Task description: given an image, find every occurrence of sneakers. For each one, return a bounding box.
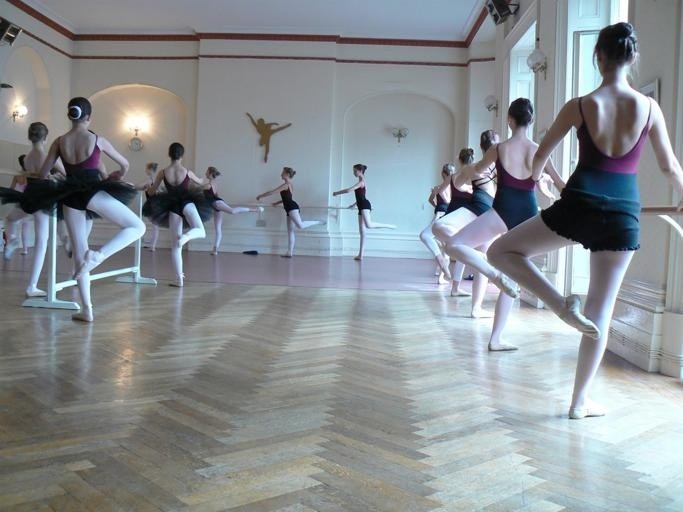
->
[436,251,606,418]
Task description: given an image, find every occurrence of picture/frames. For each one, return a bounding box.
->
[635,77,658,106]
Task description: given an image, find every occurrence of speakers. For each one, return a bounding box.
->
[485,0,511,25]
[0,18,23,47]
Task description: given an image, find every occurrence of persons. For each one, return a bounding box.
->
[331,163,396,262]
[417,95,567,352]
[254,166,327,259]
[485,21,683,422]
[0,96,165,327]
[188,165,264,255]
[143,142,207,287]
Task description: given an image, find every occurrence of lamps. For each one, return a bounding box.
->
[526,49,546,80]
[484,96,498,117]
[126,121,144,151]
[391,126,408,143]
[8,105,28,122]
